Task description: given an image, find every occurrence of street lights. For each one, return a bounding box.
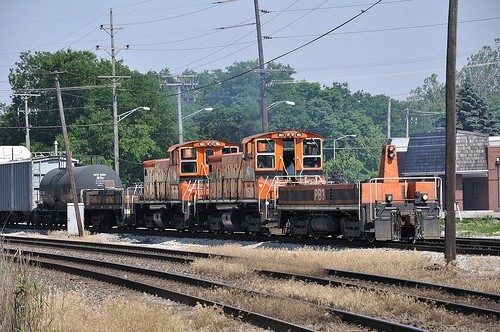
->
[178,107,214,143]
[262,100,296,131]
[114,106,150,177]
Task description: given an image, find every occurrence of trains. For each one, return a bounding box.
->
[0,128,443,243]
